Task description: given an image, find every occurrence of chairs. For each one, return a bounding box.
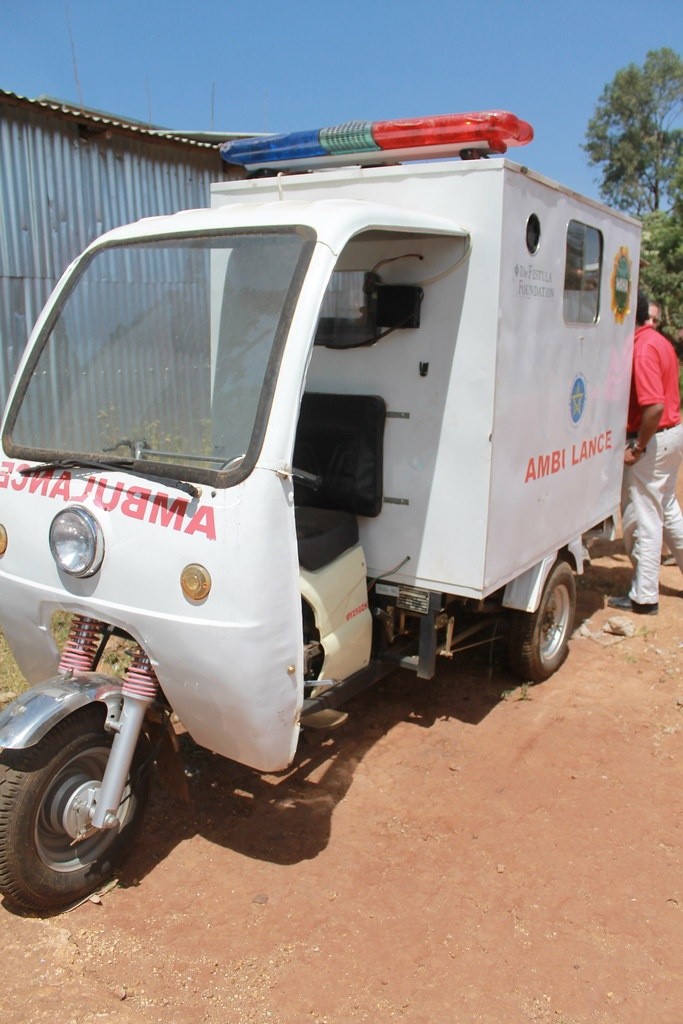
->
[292,391,385,572]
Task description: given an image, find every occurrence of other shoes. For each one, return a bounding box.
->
[661,554,677,565]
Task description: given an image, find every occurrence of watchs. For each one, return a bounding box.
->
[630,439,648,454]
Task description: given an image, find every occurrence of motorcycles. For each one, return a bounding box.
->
[0,111,644,912]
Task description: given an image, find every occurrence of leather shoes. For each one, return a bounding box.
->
[608,594,658,615]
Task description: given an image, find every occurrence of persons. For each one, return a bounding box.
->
[607,290,683,622]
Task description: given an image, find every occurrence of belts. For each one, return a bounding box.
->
[627,423,679,439]
